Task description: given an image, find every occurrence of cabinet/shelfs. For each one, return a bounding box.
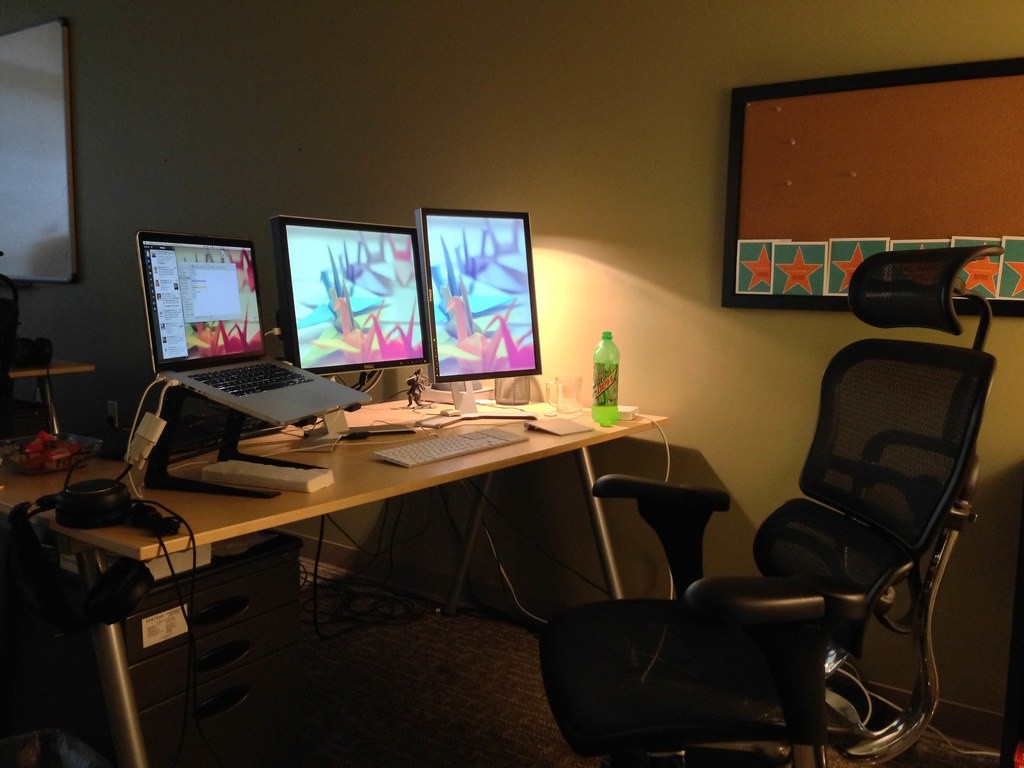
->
[0,531,309,768]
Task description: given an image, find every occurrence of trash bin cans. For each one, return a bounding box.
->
[0,727,115,768]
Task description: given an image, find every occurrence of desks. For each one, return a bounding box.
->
[0,401,671,768]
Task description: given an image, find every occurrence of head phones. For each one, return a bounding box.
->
[3,478,155,637]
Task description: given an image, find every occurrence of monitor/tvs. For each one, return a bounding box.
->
[270,215,431,452]
[415,207,542,430]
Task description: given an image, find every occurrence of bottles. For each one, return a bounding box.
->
[592,331,620,427]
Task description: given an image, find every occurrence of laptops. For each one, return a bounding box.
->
[137,230,372,426]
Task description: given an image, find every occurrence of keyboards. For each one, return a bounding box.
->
[369,429,529,468]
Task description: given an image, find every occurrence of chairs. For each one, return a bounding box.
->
[535,244,1005,768]
